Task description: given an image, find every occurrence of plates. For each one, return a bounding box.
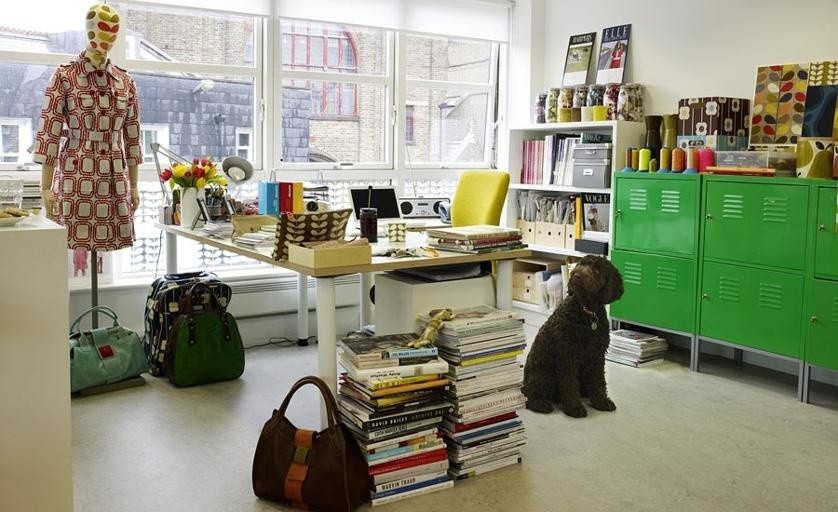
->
[0,216,25,227]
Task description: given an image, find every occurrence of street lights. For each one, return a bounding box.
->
[439,101,455,163]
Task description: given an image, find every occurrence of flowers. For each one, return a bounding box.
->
[159,154,229,198]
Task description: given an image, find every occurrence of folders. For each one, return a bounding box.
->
[293,182,304,213]
[258,181,279,217]
[283,183,293,216]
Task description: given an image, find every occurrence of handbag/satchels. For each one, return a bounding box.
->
[145,271,232,378]
[253,375,370,511]
[68,306,149,394]
[163,282,245,388]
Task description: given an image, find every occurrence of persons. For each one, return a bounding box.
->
[610,41,624,69]
[73,249,88,276]
[30,2,143,253]
[589,208,600,229]
[97,251,103,275]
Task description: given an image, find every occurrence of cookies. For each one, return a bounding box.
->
[5,207,30,217]
[0,207,18,217]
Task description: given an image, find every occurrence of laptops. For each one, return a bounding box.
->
[348,185,426,228]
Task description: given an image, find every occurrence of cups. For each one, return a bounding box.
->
[0,179,24,209]
[360,208,378,243]
[571,108,581,122]
[593,105,608,121]
[581,106,593,122]
[560,108,571,122]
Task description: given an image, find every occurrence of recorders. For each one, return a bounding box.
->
[397,195,450,218]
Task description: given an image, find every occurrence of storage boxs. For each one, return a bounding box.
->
[573,143,611,159]
[571,159,611,189]
[534,220,564,250]
[516,218,534,249]
[566,223,574,249]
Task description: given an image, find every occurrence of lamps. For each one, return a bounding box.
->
[222,156,254,186]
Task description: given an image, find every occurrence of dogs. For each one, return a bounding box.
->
[522,256,625,417]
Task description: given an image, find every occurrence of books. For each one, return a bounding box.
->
[336,332,455,507]
[604,327,669,369]
[522,136,575,187]
[427,224,528,257]
[585,196,609,232]
[534,260,577,312]
[418,304,528,482]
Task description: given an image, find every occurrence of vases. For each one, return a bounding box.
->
[179,187,206,229]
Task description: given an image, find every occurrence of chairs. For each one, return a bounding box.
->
[450,169,509,228]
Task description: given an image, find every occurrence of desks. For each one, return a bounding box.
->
[152,219,531,428]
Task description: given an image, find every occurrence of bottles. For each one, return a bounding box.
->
[535,92,546,123]
[618,84,644,121]
[557,86,574,122]
[545,88,560,123]
[586,84,606,106]
[603,83,620,120]
[574,84,588,107]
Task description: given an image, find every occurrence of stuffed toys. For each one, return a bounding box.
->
[409,307,456,350]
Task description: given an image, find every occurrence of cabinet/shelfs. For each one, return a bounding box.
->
[509,119,650,326]
[1,210,75,511]
[613,168,838,403]
